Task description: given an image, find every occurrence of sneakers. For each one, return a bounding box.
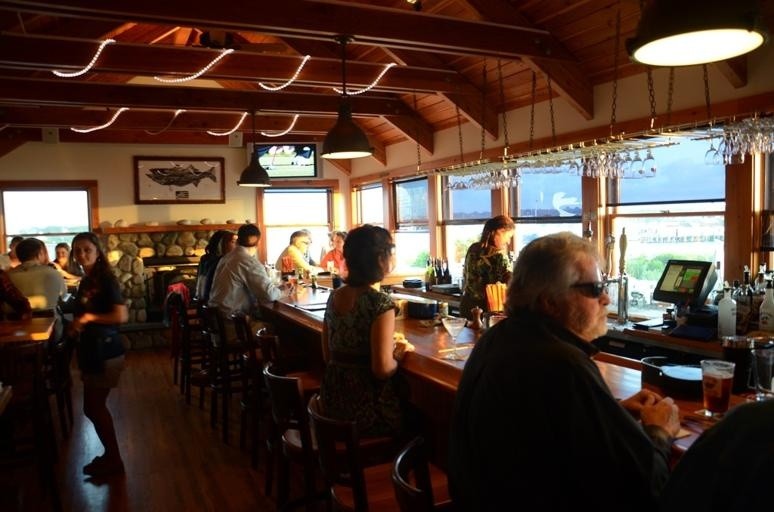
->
[83,457,125,478]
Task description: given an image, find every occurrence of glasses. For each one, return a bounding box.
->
[569,268,610,300]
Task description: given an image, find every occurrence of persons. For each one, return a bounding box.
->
[321,222,411,438]
[195,223,347,348]
[665,398,773,512]
[70,232,131,476]
[447,230,684,510]
[0,234,83,323]
[462,213,517,316]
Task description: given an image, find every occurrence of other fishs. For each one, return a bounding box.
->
[145,167,218,190]
[149,165,202,175]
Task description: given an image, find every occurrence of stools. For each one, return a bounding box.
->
[173,291,453,512]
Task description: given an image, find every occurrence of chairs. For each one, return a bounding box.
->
[1,343,55,497]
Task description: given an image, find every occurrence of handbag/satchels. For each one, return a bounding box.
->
[83,322,125,366]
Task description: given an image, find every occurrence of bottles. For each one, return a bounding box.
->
[266,263,275,281]
[424,256,450,292]
[326,259,334,271]
[716,263,774,334]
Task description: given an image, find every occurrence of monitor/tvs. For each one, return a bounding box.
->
[247,141,323,180]
[652,260,717,310]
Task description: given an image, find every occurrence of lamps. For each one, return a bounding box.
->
[319,34,373,159]
[625,1,766,66]
[236,109,272,187]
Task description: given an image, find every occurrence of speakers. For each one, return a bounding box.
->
[228,132,244,148]
[42,126,60,145]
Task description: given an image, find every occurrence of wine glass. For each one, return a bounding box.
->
[439,317,467,361]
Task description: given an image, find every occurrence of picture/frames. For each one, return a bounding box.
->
[133,156,225,204]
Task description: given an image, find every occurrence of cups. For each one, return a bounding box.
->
[288,275,298,288]
[699,336,774,416]
